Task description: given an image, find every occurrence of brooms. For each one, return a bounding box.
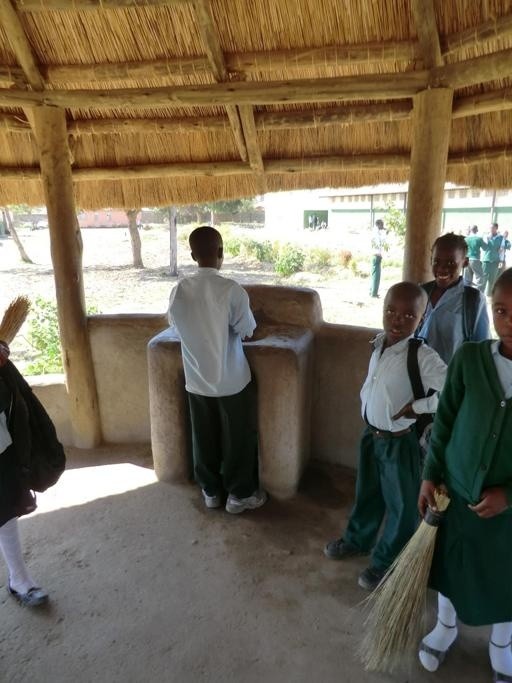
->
[357,485,452,678]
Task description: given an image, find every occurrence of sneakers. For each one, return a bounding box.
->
[201,486,268,515]
[359,564,392,590]
[5,574,50,608]
[323,534,369,558]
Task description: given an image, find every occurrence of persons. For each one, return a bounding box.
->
[309,214,329,228]
[167,227,268,513]
[418,234,490,361]
[0,340,66,607]
[462,222,512,296]
[324,282,449,590]
[369,220,386,300]
[418,268,512,681]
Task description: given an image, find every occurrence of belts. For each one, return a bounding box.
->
[364,423,412,438]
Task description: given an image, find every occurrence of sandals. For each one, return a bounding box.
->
[484,636,512,683]
[418,616,458,671]
